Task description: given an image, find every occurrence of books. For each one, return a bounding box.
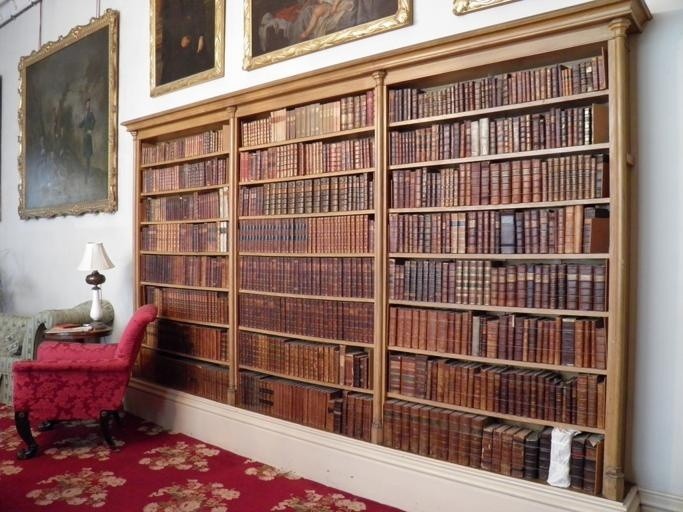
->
[142,254,228,403]
[141,124,228,251]
[384,257,607,497]
[236,255,374,444]
[236,90,378,254]
[385,46,611,254]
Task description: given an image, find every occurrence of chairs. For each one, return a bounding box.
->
[0,296,158,460]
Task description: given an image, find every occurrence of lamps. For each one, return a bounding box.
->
[75,239,115,333]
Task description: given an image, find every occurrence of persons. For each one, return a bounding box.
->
[76,96,98,182]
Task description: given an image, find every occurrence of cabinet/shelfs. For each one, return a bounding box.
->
[118,1,656,507]
